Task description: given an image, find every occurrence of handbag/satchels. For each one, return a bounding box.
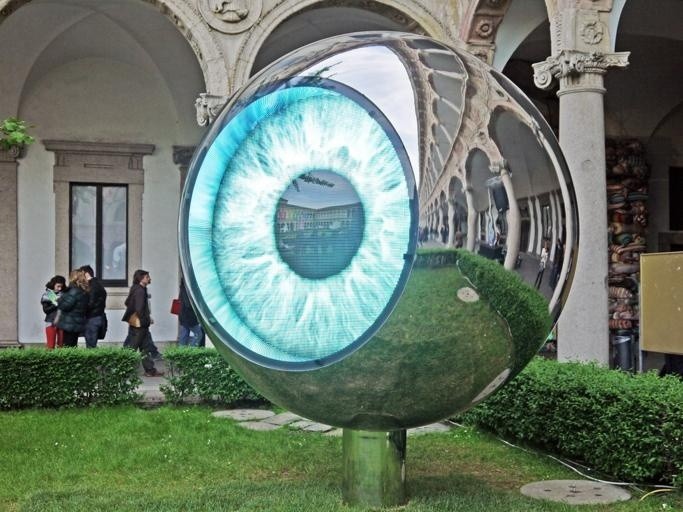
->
[171,299,182,314]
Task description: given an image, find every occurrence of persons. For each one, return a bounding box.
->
[417,224,464,249]
[534,239,550,290]
[548,239,575,297]
[40,265,108,348]
[121,270,166,377]
[144,272,164,362]
[178,278,204,348]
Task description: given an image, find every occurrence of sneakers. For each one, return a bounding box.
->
[153,353,165,361]
[144,369,165,377]
